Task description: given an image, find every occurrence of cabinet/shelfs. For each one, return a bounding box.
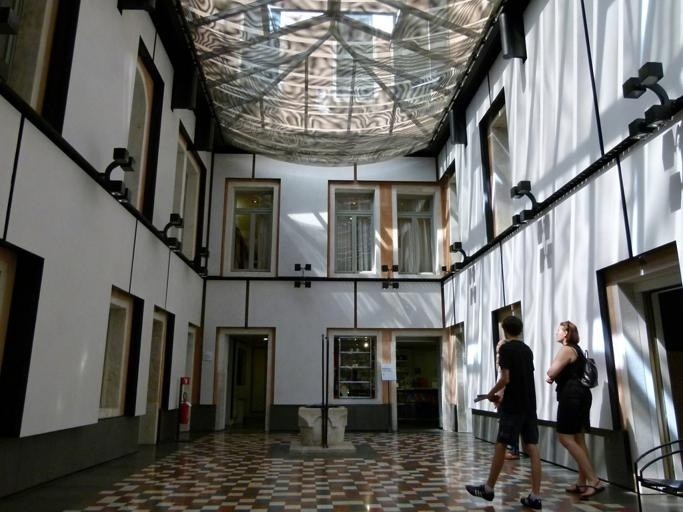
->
[338,336,373,399]
[397,348,414,377]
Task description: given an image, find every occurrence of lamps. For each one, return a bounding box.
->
[294,263,311,288]
[191,248,209,278]
[161,213,184,253]
[509,181,537,227]
[99,147,136,202]
[382,264,399,289]
[448,242,467,273]
[622,62,669,139]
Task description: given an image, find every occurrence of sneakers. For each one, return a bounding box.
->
[520,494,541,509]
[466,485,493,501]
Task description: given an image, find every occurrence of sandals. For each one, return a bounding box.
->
[566,484,583,493]
[579,479,605,500]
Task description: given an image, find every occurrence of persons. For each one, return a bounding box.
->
[465,316,542,510]
[489,340,519,460]
[546,321,605,500]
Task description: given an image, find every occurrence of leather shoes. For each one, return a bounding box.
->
[505,452,519,460]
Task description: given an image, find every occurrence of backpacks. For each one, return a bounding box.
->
[570,344,598,388]
